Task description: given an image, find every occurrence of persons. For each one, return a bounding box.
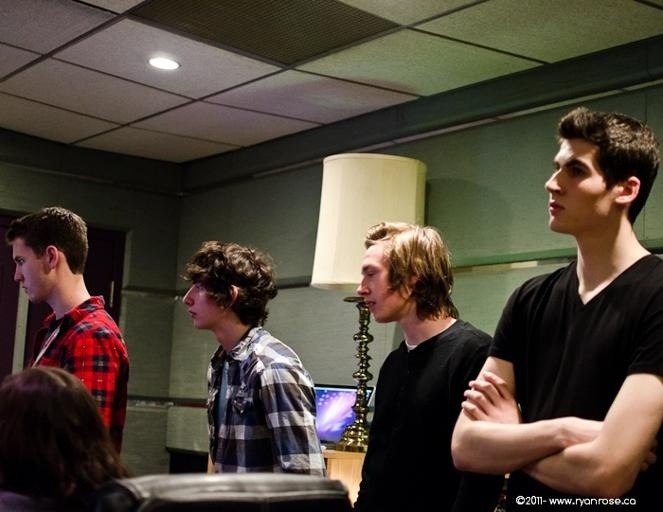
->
[450,108,663,511]
[182,241,326,477]
[8,206,129,450]
[2,370,124,512]
[353,219,505,512]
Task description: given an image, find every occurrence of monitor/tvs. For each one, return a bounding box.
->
[311,384,376,445]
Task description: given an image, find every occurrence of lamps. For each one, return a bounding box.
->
[310,152,429,452]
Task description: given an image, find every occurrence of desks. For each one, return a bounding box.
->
[319,446,366,508]
